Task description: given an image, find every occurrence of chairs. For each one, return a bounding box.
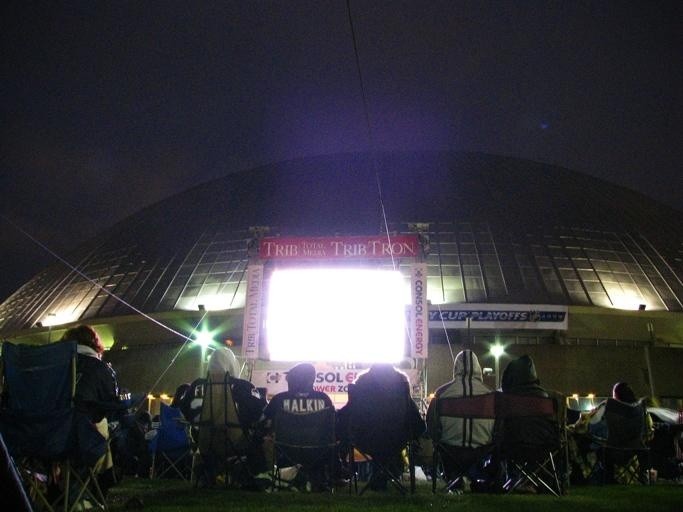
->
[2,340,112,511]
[148,385,653,495]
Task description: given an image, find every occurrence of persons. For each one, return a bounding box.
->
[56,325,133,504]
[109,346,683,497]
